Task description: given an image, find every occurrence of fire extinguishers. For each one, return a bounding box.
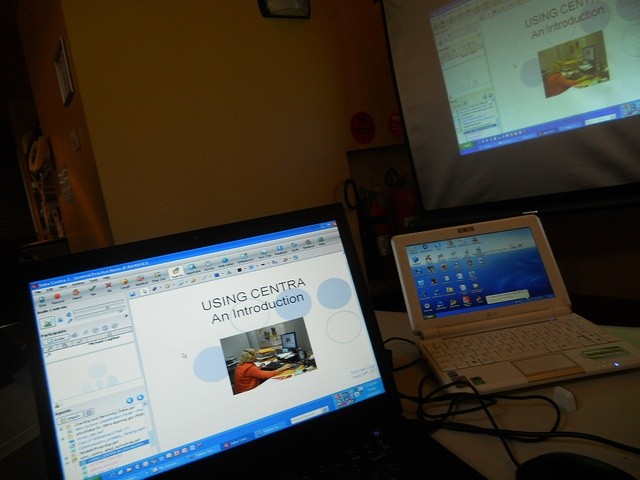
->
[385,169,424,237]
[345,179,405,311]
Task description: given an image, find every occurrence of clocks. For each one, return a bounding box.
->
[257,0,311,19]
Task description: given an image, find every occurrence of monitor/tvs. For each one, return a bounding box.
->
[383,4,639,187]
[280,332,298,351]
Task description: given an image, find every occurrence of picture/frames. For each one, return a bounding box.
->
[51,36,74,106]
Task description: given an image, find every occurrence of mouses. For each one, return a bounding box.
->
[515,452,639,480]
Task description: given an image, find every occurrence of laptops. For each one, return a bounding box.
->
[14,201,489,479]
[390,214,640,398]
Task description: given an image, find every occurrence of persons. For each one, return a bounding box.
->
[234,348,292,392]
[546,60,591,97]
